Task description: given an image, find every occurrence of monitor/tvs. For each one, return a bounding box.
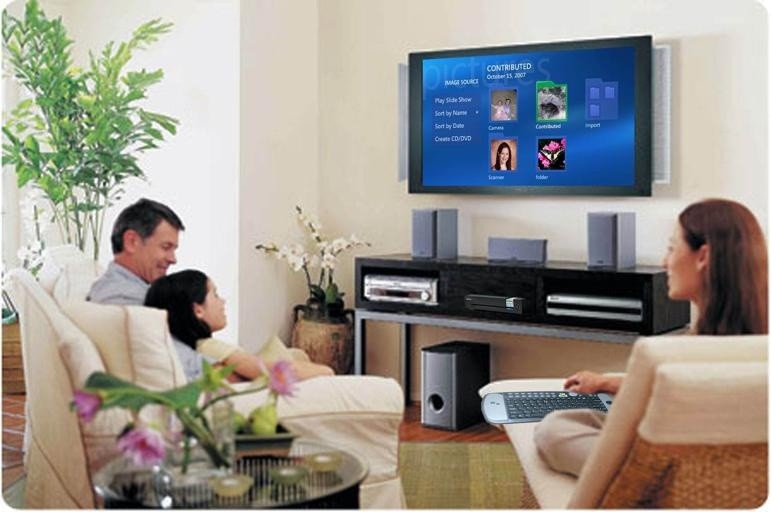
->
[408,34,653,197]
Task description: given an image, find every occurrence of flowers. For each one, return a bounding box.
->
[258,203,372,320]
[0,187,61,327]
[71,350,300,473]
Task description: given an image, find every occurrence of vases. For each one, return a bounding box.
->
[0,318,26,397]
[145,433,237,492]
[289,315,352,375]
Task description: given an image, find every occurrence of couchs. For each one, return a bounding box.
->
[0,269,414,510]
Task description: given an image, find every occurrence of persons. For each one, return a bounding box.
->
[491,141,514,171]
[502,98,515,119]
[490,100,505,119]
[540,88,560,117]
[531,196,769,480]
[143,270,336,383]
[88,197,187,308]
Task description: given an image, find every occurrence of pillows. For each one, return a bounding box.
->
[121,302,192,433]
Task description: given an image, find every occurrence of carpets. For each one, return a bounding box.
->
[1,438,540,510]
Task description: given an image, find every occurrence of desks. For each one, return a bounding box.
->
[86,436,370,508]
[347,309,699,406]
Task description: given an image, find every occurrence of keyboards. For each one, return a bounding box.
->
[481,391,613,424]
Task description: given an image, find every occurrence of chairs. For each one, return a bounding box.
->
[472,332,768,507]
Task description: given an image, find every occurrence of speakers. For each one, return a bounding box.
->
[398,63,409,181]
[421,341,490,432]
[587,212,635,269]
[487,236,547,264]
[412,209,457,259]
[652,45,673,183]
